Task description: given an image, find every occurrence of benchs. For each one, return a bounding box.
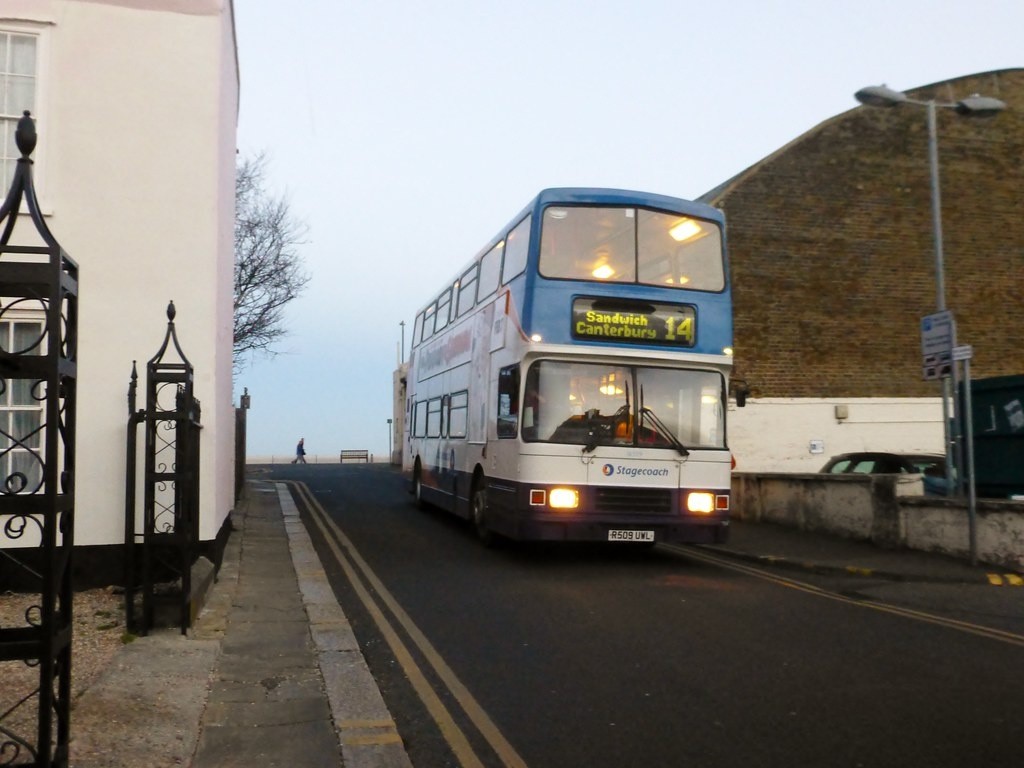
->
[340,450,368,464]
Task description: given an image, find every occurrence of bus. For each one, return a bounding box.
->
[398,185,752,545]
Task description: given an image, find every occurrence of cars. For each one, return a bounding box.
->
[819,452,991,499]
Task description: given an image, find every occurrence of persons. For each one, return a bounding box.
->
[511,385,564,431]
[292,441,308,465]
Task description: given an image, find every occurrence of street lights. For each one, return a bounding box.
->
[400,320,406,364]
[851,83,1009,497]
[387,418,393,465]
